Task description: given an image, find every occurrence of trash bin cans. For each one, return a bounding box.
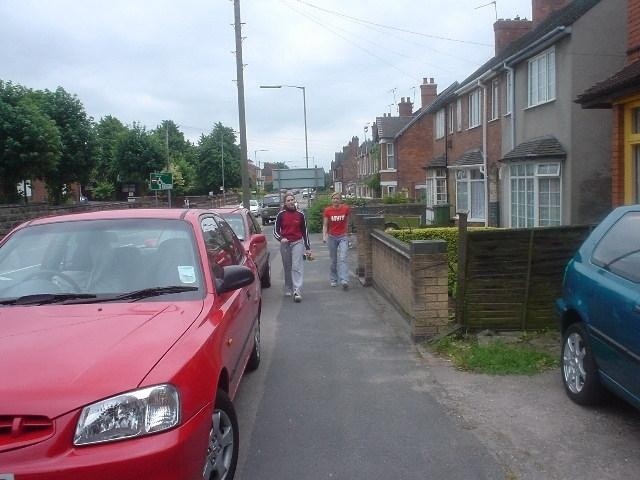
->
[432,204,453,227]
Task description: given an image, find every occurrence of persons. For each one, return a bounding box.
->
[272,193,312,303]
[321,192,352,289]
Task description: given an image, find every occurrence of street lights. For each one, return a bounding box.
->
[257,84,313,208]
[253,149,267,167]
[221,127,241,199]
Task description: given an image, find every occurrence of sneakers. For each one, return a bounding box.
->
[329,280,337,288]
[293,291,303,303]
[339,279,350,289]
[284,289,292,296]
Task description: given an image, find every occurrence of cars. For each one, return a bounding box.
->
[143,207,272,289]
[0,207,262,480]
[237,184,315,224]
[552,201,639,420]
